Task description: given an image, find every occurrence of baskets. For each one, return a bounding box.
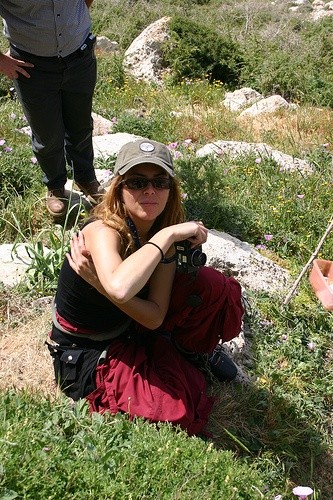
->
[308,259,333,310]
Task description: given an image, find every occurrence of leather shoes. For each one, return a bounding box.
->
[44,177,107,217]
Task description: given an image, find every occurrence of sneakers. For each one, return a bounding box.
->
[198,344,239,386]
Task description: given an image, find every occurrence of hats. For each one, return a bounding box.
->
[113,138,174,177]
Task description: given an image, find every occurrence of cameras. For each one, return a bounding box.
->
[175,240,207,274]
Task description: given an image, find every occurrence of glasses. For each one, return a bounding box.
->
[121,171,176,191]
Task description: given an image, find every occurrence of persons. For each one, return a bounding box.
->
[44,139,245,436]
[0,0,107,216]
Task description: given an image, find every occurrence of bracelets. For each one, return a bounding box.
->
[144,242,165,260]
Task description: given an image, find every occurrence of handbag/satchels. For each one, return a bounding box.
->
[49,343,104,402]
